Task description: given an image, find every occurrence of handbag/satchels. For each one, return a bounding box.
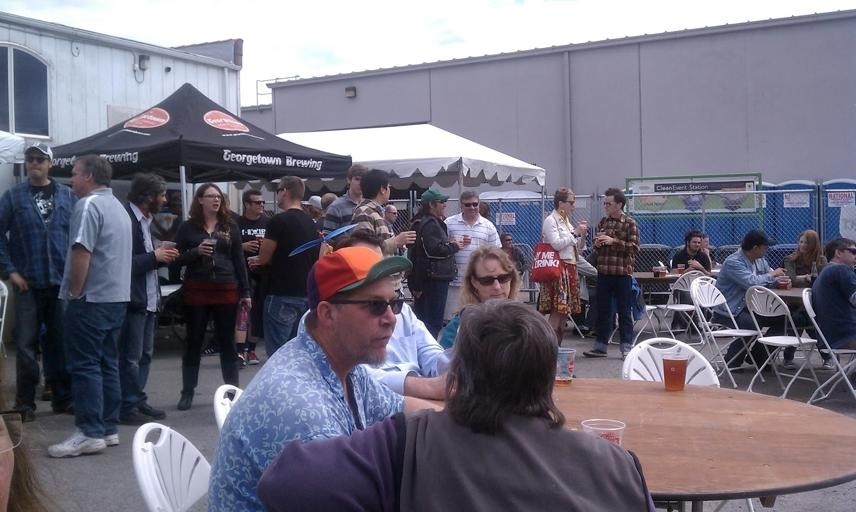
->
[571,232,578,239]
[581,234,587,240]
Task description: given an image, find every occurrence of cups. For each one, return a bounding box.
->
[603,201,620,207]
[386,211,398,215]
[562,200,575,205]
[201,194,223,200]
[432,199,447,204]
[248,198,266,205]
[25,156,51,163]
[461,201,480,207]
[1,410,25,455]
[838,247,856,255]
[472,272,512,286]
[328,295,405,315]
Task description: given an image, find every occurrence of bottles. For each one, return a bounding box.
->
[23,142,54,161]
[300,196,322,211]
[305,245,414,310]
[743,229,777,246]
[421,189,450,206]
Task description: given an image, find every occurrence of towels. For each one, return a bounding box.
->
[719,244,741,263]
[674,243,717,264]
[769,243,799,269]
[634,243,673,304]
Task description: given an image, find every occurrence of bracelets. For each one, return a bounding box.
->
[653,267,667,277]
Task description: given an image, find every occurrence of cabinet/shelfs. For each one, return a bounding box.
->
[235,302,249,344]
[810,261,818,285]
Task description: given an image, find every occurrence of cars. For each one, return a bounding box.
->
[677,264,686,274]
[582,419,625,447]
[163,241,177,248]
[779,275,789,288]
[555,348,576,385]
[578,221,589,226]
[664,351,689,392]
[203,239,217,256]
[455,235,463,243]
[596,232,606,238]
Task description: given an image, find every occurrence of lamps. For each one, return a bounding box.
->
[622,337,754,512]
[213,385,244,431]
[607,274,662,348]
[802,287,856,405]
[534,281,585,338]
[689,276,736,339]
[0,280,8,345]
[512,244,534,289]
[745,286,825,399]
[656,270,706,346]
[132,421,211,511]
[694,281,765,388]
[658,259,678,318]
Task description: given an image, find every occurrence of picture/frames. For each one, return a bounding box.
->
[427,377,856,512]
[629,271,682,304]
[763,287,811,336]
[152,284,183,353]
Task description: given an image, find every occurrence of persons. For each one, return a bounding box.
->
[1,408,63,512]
[351,169,417,256]
[167,181,252,411]
[300,195,323,233]
[322,193,338,211]
[582,186,640,360]
[119,172,181,427]
[234,189,273,369]
[255,298,657,512]
[500,232,528,281]
[701,232,724,270]
[421,185,465,341]
[406,210,423,321]
[443,191,502,328]
[0,142,80,424]
[670,231,712,336]
[380,204,398,238]
[322,163,370,241]
[207,244,448,511]
[47,154,134,459]
[573,254,598,337]
[811,238,856,350]
[542,187,589,348]
[247,175,321,362]
[296,228,458,402]
[713,229,784,374]
[784,230,837,370]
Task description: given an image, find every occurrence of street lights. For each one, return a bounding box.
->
[422,253,459,281]
[528,243,565,283]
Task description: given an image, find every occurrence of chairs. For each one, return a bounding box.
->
[46,429,108,458]
[52,402,75,415]
[14,405,35,422]
[619,351,631,362]
[200,345,221,356]
[782,359,796,370]
[137,401,166,421]
[237,352,247,369]
[246,350,261,365]
[822,356,836,370]
[41,384,53,402]
[117,405,154,426]
[582,347,608,358]
[177,389,196,410]
[104,431,120,446]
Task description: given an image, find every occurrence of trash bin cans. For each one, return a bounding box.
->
[66,291,85,301]
